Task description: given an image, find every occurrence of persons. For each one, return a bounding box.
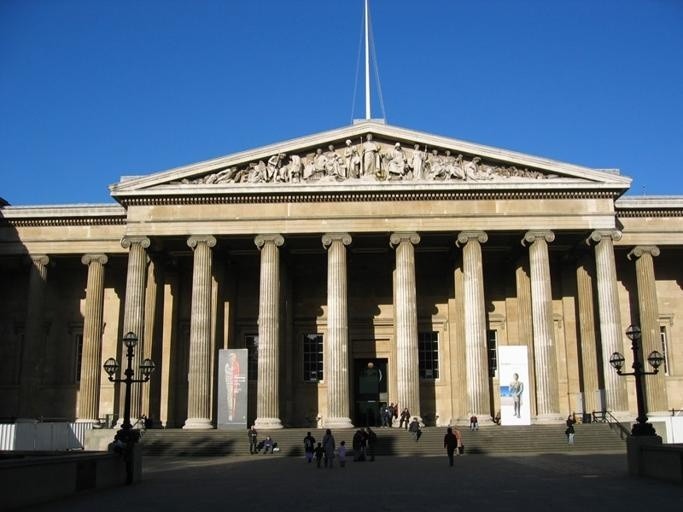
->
[509,373,524,418]
[453,428,465,456]
[470,414,479,430]
[565,414,578,448]
[399,408,410,431]
[247,424,258,455]
[380,402,391,431]
[303,427,377,469]
[260,436,275,456]
[443,427,457,467]
[407,417,422,440]
[495,409,502,424]
[224,352,240,424]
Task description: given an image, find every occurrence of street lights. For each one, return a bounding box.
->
[104,332,158,483]
[608,322,664,436]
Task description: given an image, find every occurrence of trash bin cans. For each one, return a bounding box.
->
[583,414,591,423]
